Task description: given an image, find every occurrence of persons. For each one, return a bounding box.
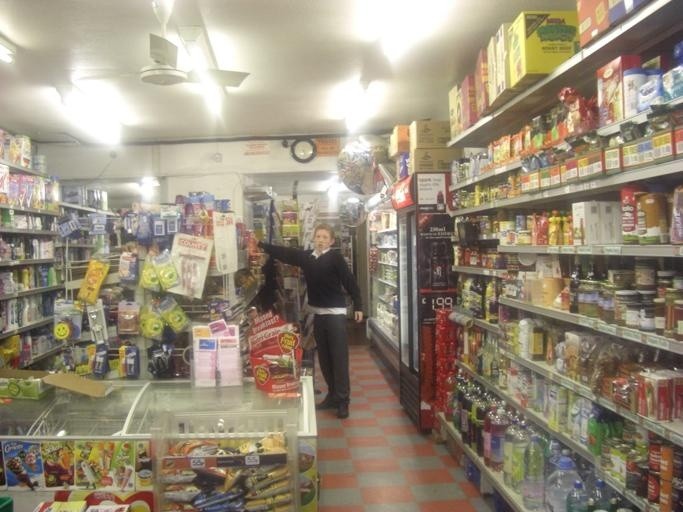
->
[253,224,363,419]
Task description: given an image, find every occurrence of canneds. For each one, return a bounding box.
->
[578,256,683,344]
[646,437,683,512]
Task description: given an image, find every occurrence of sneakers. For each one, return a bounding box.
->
[316,395,338,409]
[337,402,348,418]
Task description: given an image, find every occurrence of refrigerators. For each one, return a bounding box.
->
[389,171,456,438]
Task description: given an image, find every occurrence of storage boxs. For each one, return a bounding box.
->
[447,84,463,140]
[487,23,527,108]
[475,48,498,117]
[388,124,410,160]
[409,118,462,152]
[608,0,653,27]
[409,148,462,175]
[462,74,478,131]
[507,11,582,89]
[396,153,410,182]
[576,0,616,49]
[390,174,415,211]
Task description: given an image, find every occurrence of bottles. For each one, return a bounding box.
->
[468,276,498,322]
[546,209,569,247]
[436,190,444,213]
[576,256,682,342]
[443,369,608,512]
[429,241,448,290]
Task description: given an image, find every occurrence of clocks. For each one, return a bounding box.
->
[291,138,318,163]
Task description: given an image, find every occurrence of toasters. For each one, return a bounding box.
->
[568,263,584,312]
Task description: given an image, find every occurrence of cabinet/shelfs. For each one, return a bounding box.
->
[55,202,122,348]
[1,157,61,369]
[434,0,682,511]
[364,185,399,374]
[244,186,354,383]
[120,230,270,380]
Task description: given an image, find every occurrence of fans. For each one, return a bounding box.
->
[65,1,251,89]
